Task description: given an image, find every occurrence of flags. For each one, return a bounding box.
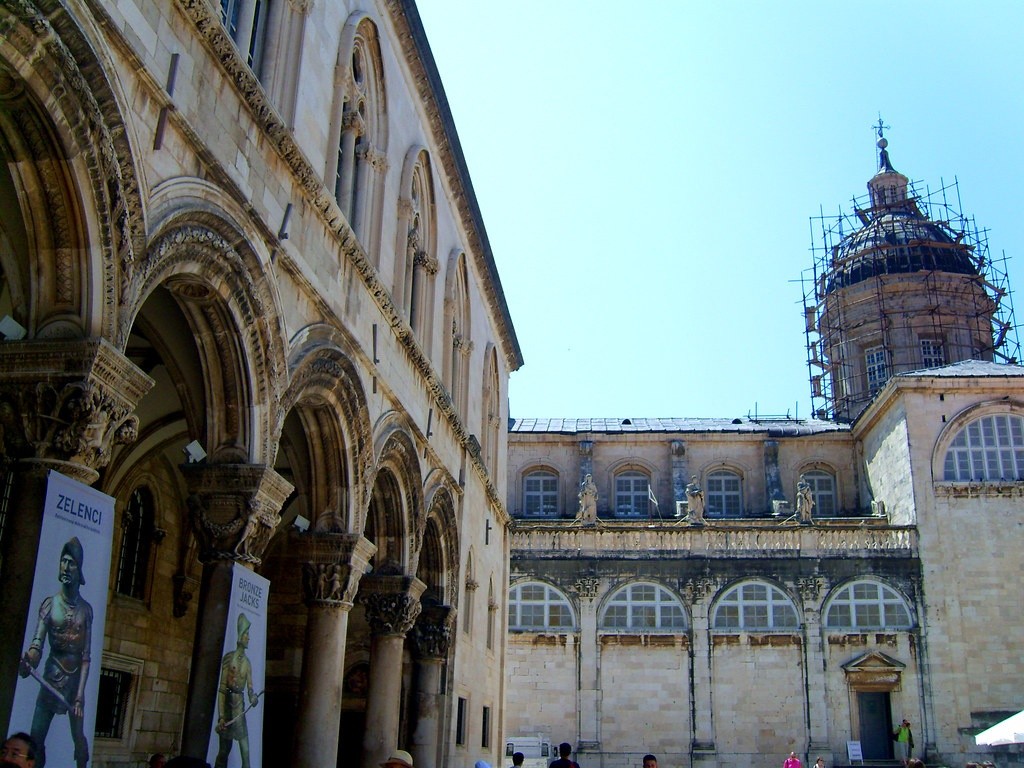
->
[649,489,658,505]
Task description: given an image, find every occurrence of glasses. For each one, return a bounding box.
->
[0,750,33,760]
[819,760,823,761]
[904,723,906,724]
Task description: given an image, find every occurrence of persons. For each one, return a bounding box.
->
[215,613,258,768]
[797,475,812,522]
[149,753,165,768]
[379,750,414,768]
[966,761,996,768]
[20,537,94,768]
[813,755,827,768]
[684,475,705,523]
[643,755,657,768]
[894,719,914,767]
[578,474,598,524]
[783,752,803,768]
[475,760,492,768]
[0,732,44,768]
[549,743,580,768]
[906,758,926,768]
[510,752,525,768]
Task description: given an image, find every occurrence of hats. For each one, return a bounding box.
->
[378,750,414,768]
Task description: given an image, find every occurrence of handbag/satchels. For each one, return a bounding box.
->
[893,725,901,741]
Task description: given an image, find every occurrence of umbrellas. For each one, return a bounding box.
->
[975,710,1024,746]
